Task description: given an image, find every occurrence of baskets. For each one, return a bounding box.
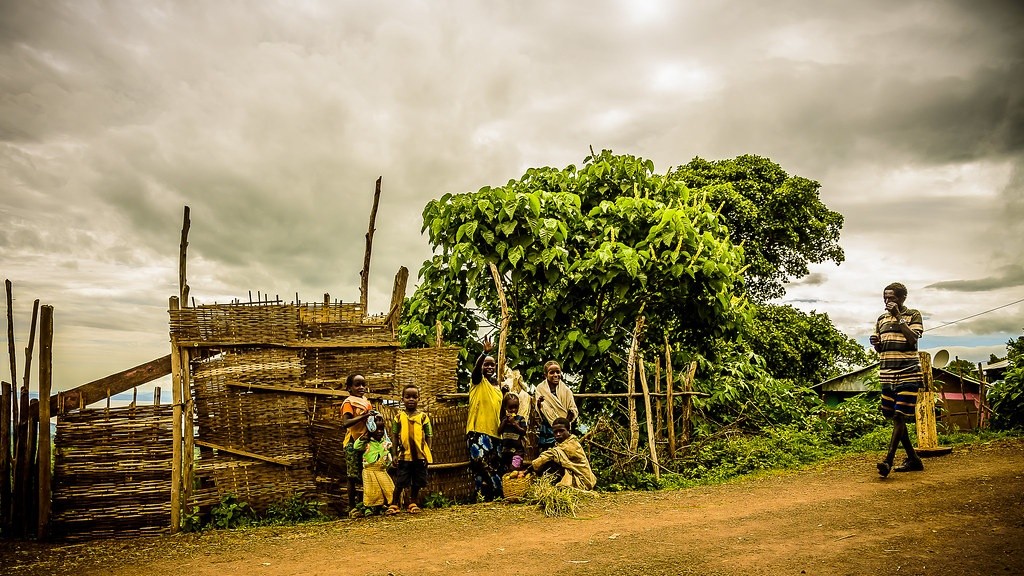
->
[501,473,532,500]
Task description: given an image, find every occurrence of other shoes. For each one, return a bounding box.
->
[894,457,922,472]
[877,461,892,477]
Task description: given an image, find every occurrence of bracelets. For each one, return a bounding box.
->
[482,350,490,356]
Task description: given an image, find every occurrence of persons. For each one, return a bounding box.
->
[518,417,597,491]
[870,282,925,477]
[353,410,402,517]
[532,360,579,480]
[385,384,433,514]
[497,391,528,474]
[466,335,504,503]
[341,373,377,520]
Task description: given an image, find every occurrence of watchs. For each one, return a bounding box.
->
[899,319,906,325]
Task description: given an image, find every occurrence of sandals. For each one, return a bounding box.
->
[364,508,372,516]
[406,503,421,513]
[348,508,362,518]
[384,506,400,515]
